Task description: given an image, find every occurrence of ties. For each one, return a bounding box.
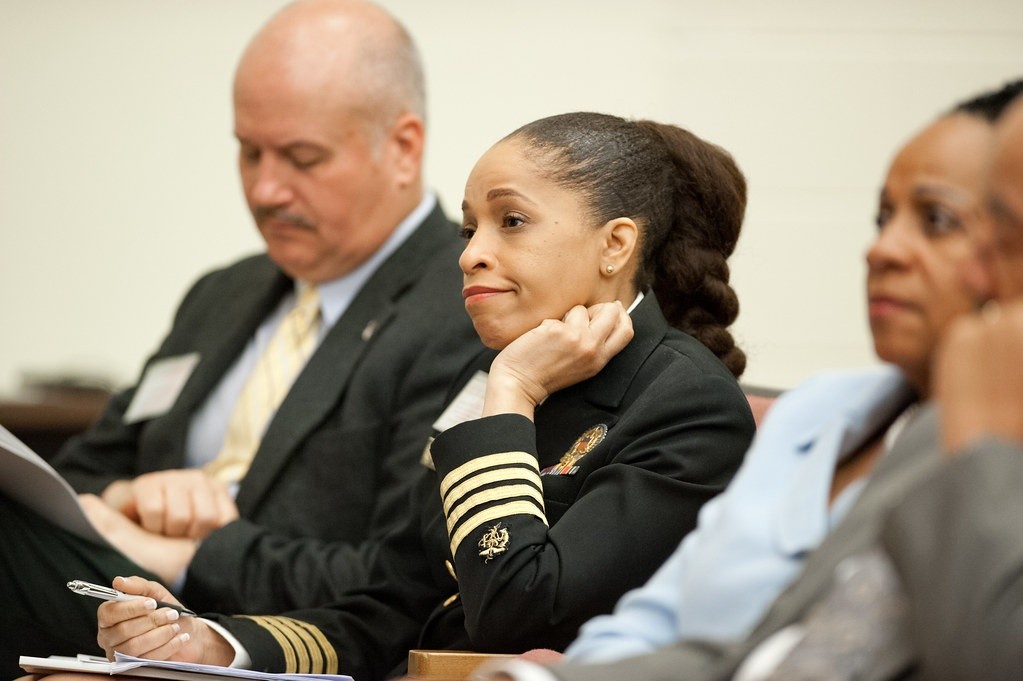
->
[202,284,322,490]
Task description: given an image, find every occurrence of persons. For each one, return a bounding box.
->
[0,0,487,681]
[490,78,1023,681]
[96,113,756,681]
[518,93,998,664]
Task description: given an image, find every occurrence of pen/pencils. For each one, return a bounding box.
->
[67,579,199,618]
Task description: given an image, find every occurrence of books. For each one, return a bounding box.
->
[16,654,353,681]
